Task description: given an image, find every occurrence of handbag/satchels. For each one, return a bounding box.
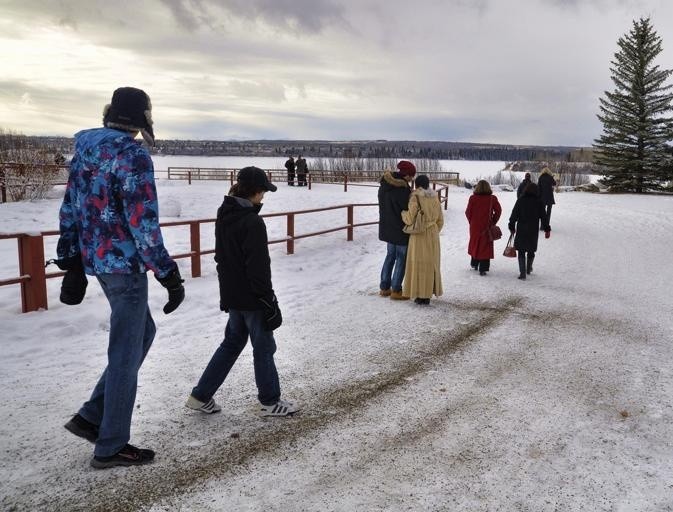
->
[503,246,517,257]
[402,210,423,233]
[488,225,502,241]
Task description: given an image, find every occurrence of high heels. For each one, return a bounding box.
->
[470,263,478,270]
[527,268,532,274]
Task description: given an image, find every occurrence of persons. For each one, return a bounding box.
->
[185,165,299,418]
[465,179,502,275]
[56,85,185,468]
[538,166,556,230]
[400,175,444,305]
[377,161,416,300]
[285,154,308,186]
[509,183,552,279]
[517,173,537,199]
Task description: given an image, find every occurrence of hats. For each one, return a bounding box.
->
[103,87,155,146]
[237,166,277,192]
[397,161,416,177]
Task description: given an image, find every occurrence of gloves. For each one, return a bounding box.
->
[153,261,186,315]
[54,250,87,305]
[258,293,282,331]
[545,230,550,239]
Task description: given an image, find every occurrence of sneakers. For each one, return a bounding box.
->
[380,289,391,296]
[185,395,221,414]
[257,401,299,417]
[391,290,410,300]
[64,414,100,444]
[90,444,155,468]
